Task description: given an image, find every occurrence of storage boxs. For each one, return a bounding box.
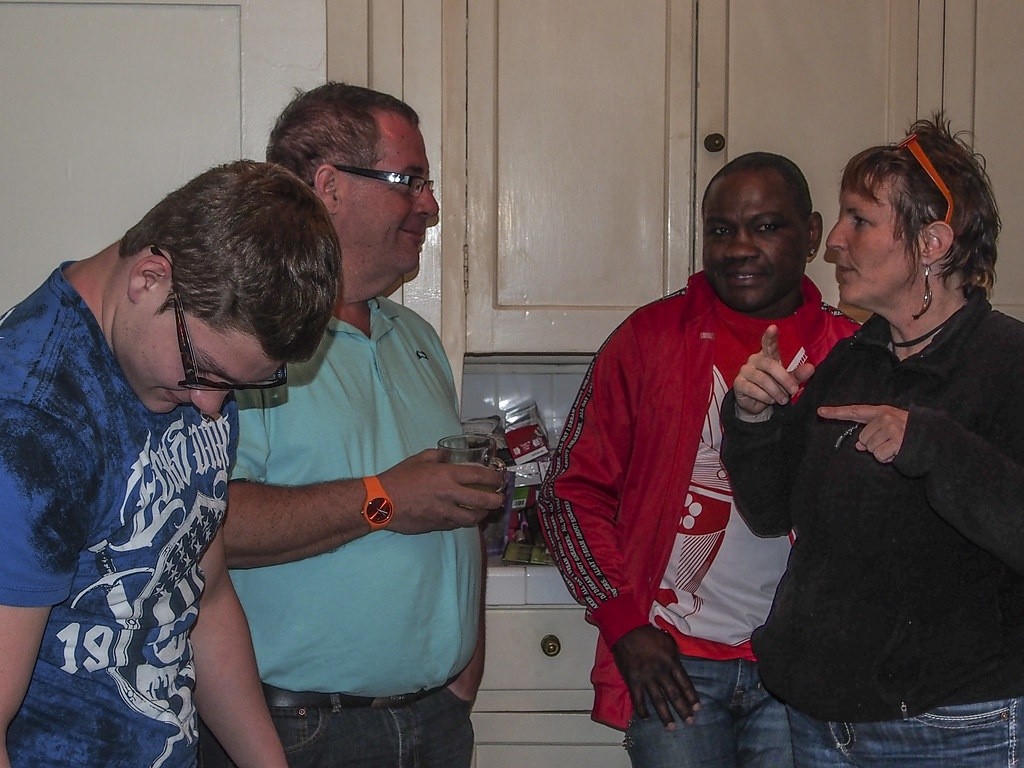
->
[505,450,559,565]
[503,400,550,465]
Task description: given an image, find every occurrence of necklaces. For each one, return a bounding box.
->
[888,307,964,349]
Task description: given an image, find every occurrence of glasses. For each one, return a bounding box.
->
[151,245,288,390]
[897,132,954,228]
[308,164,435,197]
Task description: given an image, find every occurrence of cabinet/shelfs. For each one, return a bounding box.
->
[464,0,1024,375]
[469,601,632,768]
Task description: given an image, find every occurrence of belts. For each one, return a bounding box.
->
[260,678,457,709]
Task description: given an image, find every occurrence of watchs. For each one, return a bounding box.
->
[361,475,396,532]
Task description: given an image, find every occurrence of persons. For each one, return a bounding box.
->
[722,108,1023,768]
[1,157,342,768]
[199,78,508,768]
[536,149,859,768]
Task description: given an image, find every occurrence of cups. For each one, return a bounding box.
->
[436,434,510,511]
